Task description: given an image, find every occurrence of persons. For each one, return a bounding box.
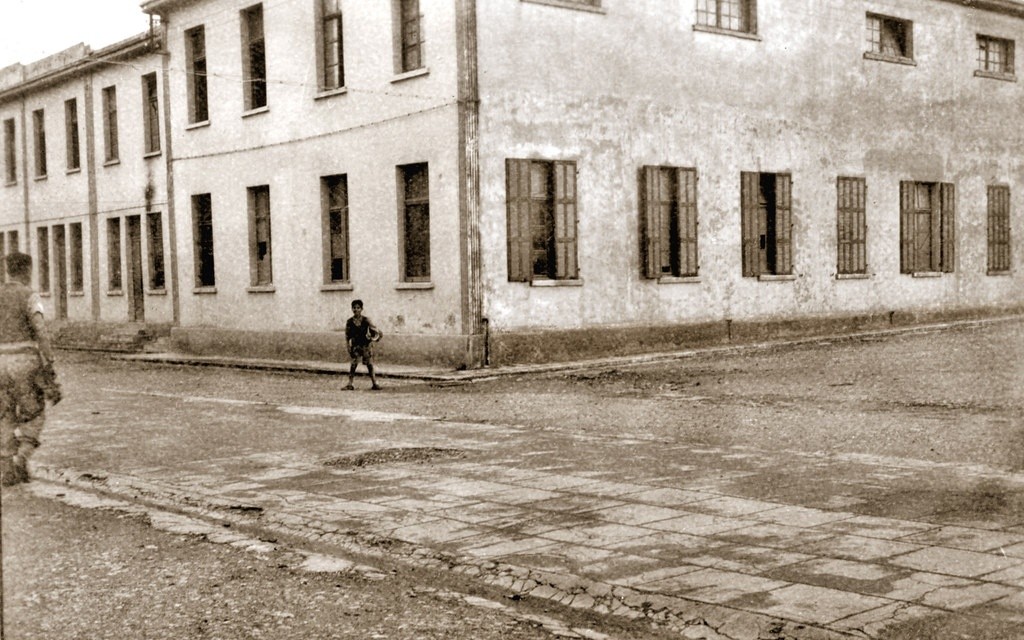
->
[342,300,383,390]
[0,252,61,486]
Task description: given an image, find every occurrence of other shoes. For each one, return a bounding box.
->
[4,474,20,486]
[373,383,382,390]
[12,456,32,482]
[341,385,354,390]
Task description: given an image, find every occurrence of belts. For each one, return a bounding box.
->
[0,346,40,355]
